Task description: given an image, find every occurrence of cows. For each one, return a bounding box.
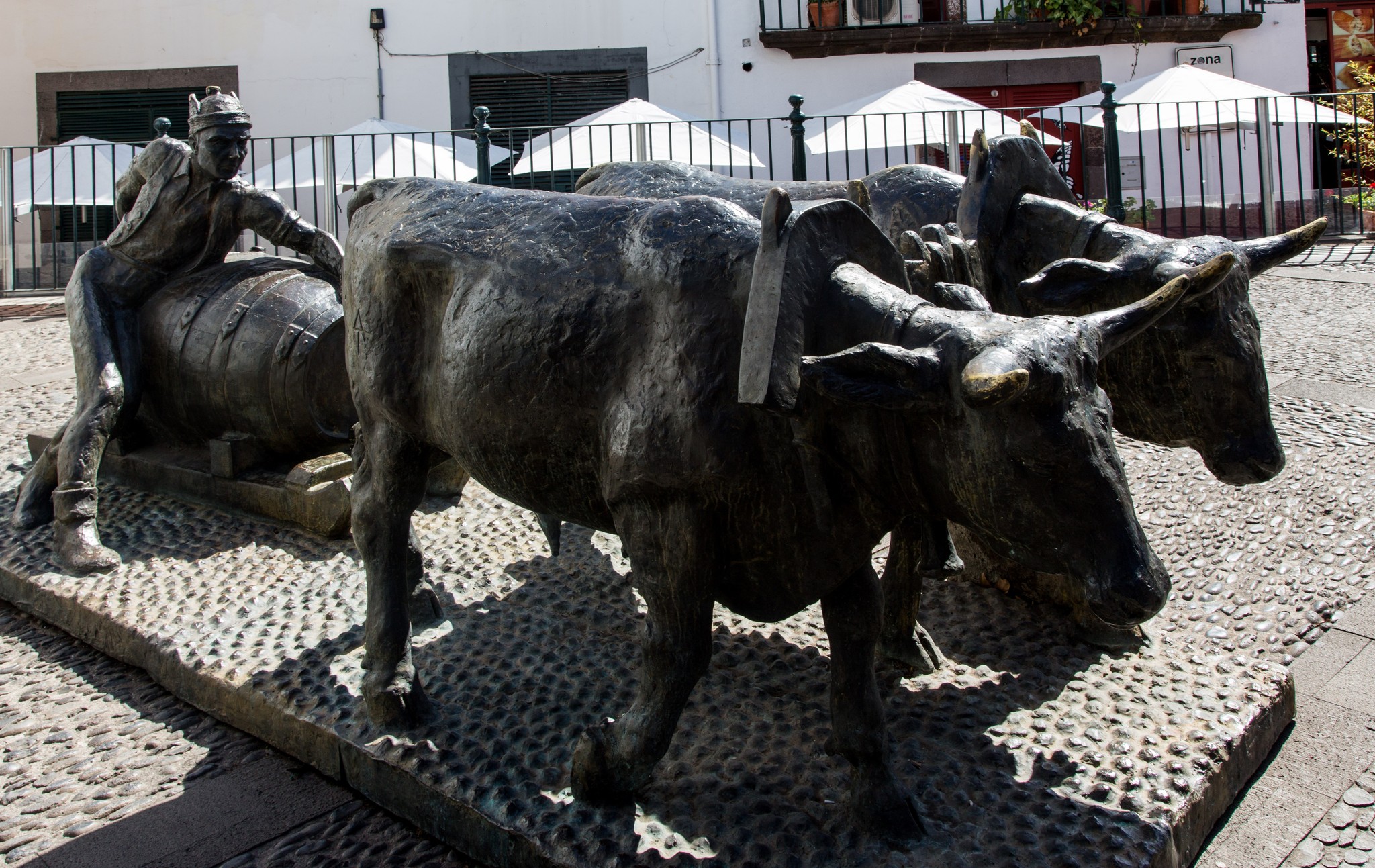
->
[565,160,1330,674]
[335,180,1191,840]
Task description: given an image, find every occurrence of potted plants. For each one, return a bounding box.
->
[808,0,843,30]
[993,0,1103,37]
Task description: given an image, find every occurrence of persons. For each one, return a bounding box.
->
[14,84,344,575]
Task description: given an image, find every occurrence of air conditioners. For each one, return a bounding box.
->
[846,0,923,27]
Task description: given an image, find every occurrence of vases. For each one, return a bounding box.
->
[1119,220,1150,232]
[1178,0,1204,16]
[1357,207,1375,231]
[1125,0,1150,17]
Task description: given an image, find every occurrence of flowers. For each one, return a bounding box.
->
[1323,183,1375,211]
[1077,195,1158,224]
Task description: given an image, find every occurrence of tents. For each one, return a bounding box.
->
[507,98,766,176]
[786,79,1070,156]
[0,136,150,208]
[236,118,517,190]
[1026,62,1371,133]
